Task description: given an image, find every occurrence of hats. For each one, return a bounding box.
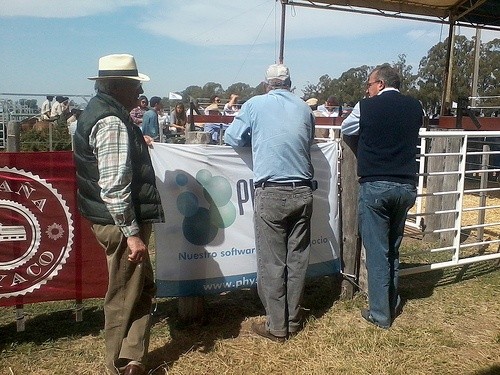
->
[86,54,150,81]
[266,64,290,84]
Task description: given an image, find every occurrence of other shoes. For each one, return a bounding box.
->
[251,321,297,342]
[360,308,391,329]
[124,365,145,375]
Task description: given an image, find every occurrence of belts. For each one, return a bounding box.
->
[256,180,311,187]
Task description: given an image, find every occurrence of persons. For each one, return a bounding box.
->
[141,96,161,142]
[170,103,186,130]
[130,96,149,128]
[67,108,78,151]
[341,67,426,330]
[73,55,166,375]
[41,96,70,119]
[313,96,343,117]
[224,63,316,341]
[158,110,165,143]
[204,95,222,140]
[221,94,241,133]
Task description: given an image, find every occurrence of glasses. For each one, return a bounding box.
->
[136,83,142,89]
[142,101,144,103]
[368,80,381,87]
[217,99,219,102]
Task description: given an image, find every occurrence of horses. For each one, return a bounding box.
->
[18,105,70,136]
[32,108,83,134]
